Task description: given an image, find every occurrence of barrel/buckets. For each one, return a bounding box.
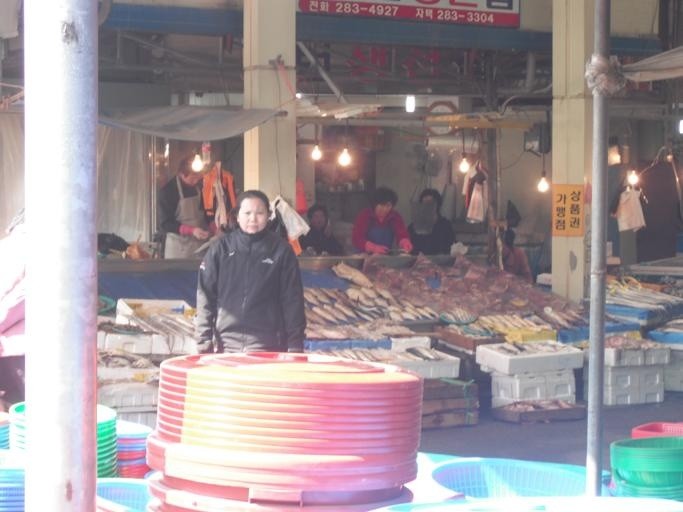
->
[608,436,683,500]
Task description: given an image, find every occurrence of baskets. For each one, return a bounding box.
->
[0,401,151,508]
[609,422,683,499]
[430,456,608,502]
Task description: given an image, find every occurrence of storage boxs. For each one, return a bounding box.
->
[491,363,664,412]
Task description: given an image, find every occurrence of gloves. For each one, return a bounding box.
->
[364,242,389,255]
[399,239,413,252]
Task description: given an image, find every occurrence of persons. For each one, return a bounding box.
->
[156,153,215,260]
[494,229,533,285]
[298,204,342,256]
[352,185,414,255]
[407,188,457,257]
[194,189,307,355]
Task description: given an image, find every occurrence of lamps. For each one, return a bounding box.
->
[459,127,471,174]
[536,127,549,193]
[311,125,322,161]
[626,125,640,185]
[192,124,204,173]
[336,126,352,167]
